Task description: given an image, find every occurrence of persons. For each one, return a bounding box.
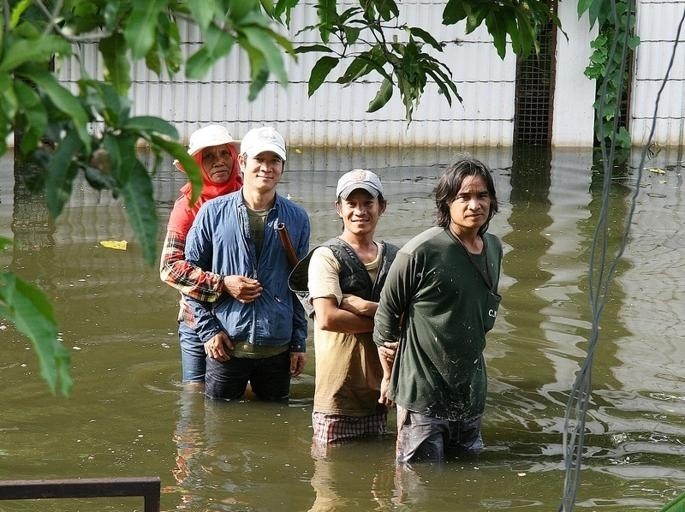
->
[158,124,265,384]
[305,166,404,445]
[181,126,310,405]
[370,157,505,468]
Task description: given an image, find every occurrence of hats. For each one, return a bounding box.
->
[171,124,242,173]
[336,169,385,202]
[240,126,287,161]
